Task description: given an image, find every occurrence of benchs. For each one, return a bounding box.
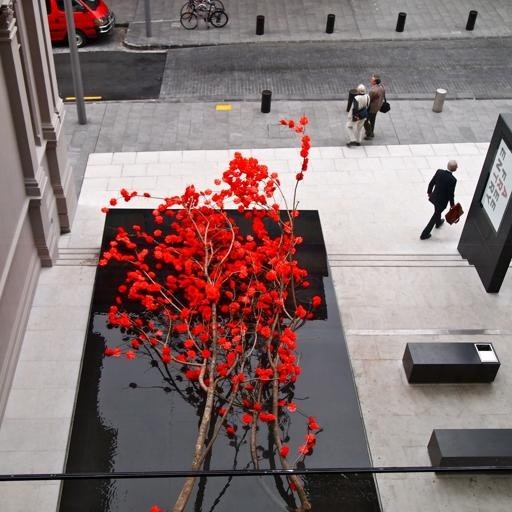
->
[401,341,500,385]
[428,428,512,476]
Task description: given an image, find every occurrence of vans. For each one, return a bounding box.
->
[45,0,115,48]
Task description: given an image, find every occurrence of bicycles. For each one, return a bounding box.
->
[180,0,228,30]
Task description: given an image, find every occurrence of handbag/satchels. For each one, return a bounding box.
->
[445,202,464,225]
[353,109,369,121]
[380,102,391,113]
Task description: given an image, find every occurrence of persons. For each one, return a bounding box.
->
[420,159,459,240]
[344,74,385,145]
